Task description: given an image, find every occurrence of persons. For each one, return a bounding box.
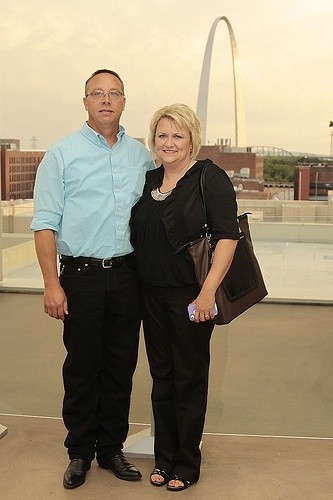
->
[29,69,157,490]
[128,102,241,492]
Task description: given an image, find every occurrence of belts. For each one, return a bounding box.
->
[61,254,135,270]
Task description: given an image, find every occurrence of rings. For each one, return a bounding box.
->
[205,315,209,318]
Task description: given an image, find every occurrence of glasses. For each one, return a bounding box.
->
[86,90,124,100]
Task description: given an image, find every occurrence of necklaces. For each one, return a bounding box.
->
[151,161,197,201]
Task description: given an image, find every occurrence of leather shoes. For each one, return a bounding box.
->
[63,458,93,489]
[96,453,142,480]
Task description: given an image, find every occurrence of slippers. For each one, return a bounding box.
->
[149,468,170,487]
[166,474,192,491]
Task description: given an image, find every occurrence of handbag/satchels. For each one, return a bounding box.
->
[187,163,268,326]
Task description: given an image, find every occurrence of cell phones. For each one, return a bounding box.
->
[187,303,218,321]
